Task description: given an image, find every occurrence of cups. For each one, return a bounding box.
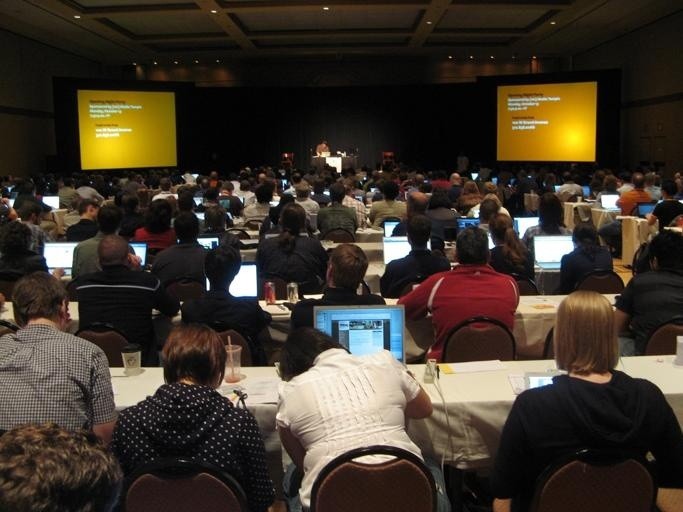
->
[223,344,244,383]
[119,342,142,377]
[576,196,582,203]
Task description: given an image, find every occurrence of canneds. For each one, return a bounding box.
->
[263,280,276,305]
[286,282,299,304]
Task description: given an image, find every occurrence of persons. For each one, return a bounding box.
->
[0,168,683,364]
[315,140,330,156]
[2,423,128,510]
[276,329,454,510]
[2,272,116,451]
[115,321,276,511]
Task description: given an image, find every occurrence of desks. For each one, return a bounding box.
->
[0,193,683,512]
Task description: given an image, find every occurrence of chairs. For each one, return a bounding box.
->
[382,151,395,166]
[281,152,295,167]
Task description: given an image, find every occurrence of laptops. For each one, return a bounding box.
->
[2,166,579,304]
[600,193,619,210]
[523,371,564,390]
[636,201,655,218]
[313,305,406,365]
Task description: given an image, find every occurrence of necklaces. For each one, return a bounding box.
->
[486,290,683,510]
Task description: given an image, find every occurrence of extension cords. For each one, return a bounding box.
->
[425,359,436,380]
